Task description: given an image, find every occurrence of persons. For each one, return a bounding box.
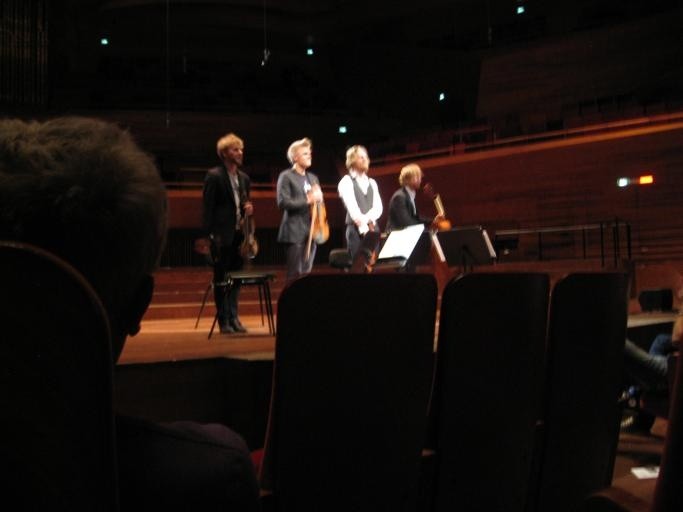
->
[0,112,264,510]
[617,335,683,436]
[336,142,384,267]
[619,278,683,404]
[273,137,325,279]
[203,133,255,335]
[385,163,444,235]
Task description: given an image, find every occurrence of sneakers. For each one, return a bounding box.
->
[219,324,235,334]
[229,319,248,332]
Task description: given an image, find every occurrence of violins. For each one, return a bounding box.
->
[239,191,258,259]
[310,181,329,244]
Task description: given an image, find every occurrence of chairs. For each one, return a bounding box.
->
[259,268,438,504]
[429,267,553,509]
[528,269,625,505]
[327,248,353,267]
[4,244,122,510]
[208,243,275,341]
